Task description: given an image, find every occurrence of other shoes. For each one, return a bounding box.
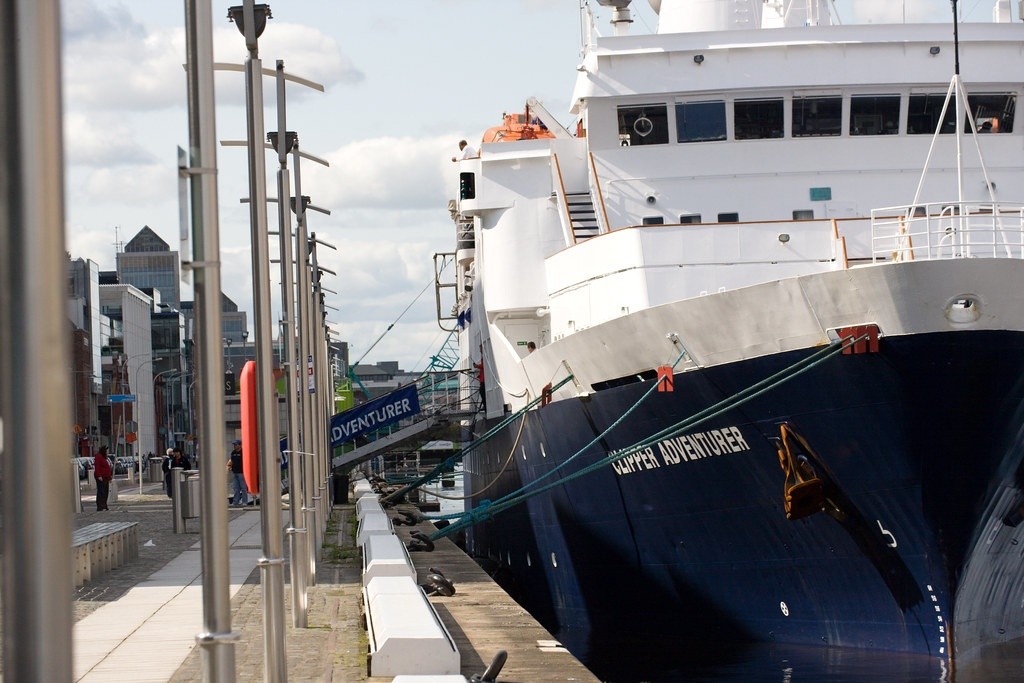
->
[242,503,247,506]
[229,504,234,506]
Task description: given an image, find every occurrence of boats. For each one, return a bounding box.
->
[433,0,1024,683]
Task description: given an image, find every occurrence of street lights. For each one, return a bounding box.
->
[121,353,189,454]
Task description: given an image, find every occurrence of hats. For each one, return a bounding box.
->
[232,440,241,444]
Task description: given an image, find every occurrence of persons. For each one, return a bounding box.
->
[94,444,114,511]
[452,140,478,163]
[162,440,248,506]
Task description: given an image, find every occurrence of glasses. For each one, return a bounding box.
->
[234,444,237,446]
